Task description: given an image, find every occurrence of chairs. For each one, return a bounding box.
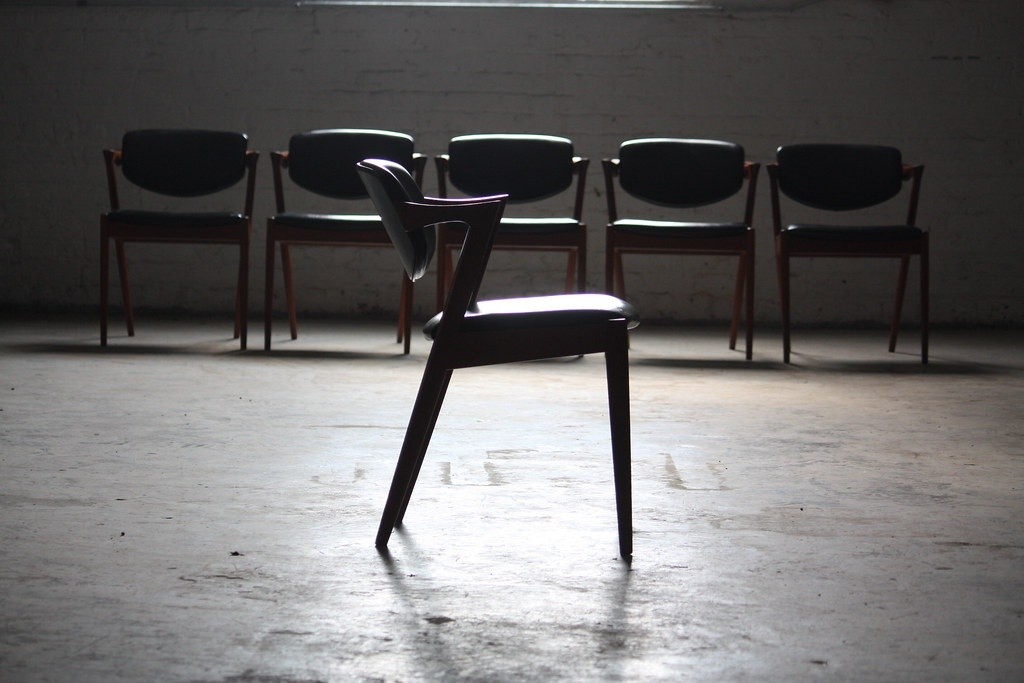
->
[97,129,260,351]
[433,136,590,358]
[766,143,930,365]
[265,129,428,354]
[601,138,762,361]
[355,159,642,558]
